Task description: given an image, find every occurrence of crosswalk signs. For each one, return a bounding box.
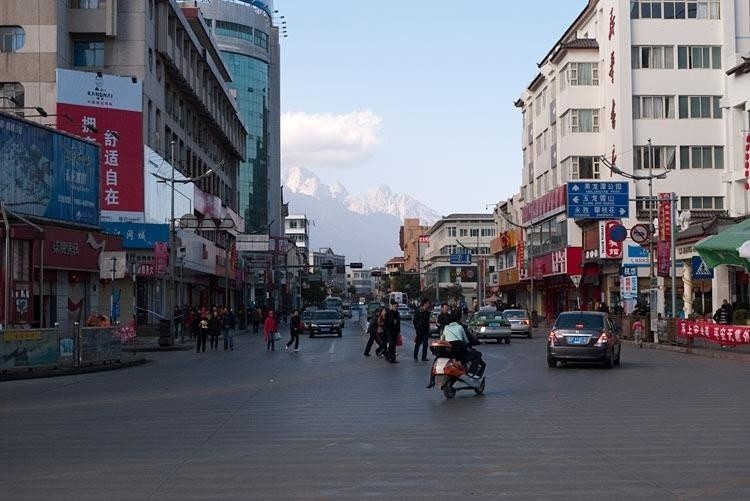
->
[692,256,714,280]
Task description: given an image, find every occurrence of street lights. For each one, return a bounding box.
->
[152,141,226,323]
[458,230,491,312]
[599,138,679,344]
[500,211,557,313]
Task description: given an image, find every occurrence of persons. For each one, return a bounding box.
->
[722,299,734,325]
[169,299,303,355]
[594,299,649,351]
[712,305,732,351]
[361,297,483,388]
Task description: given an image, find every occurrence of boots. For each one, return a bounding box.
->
[425,375,436,390]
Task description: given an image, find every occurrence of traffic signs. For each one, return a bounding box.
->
[568,180,627,193]
[569,206,628,218]
[568,194,628,206]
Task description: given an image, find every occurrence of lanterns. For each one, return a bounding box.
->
[742,134,750,191]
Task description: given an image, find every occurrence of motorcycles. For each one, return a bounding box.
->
[429,335,487,400]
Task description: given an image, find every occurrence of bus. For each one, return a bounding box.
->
[382,291,409,310]
[323,297,342,312]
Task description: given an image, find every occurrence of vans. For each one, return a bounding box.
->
[365,303,381,321]
[342,303,352,318]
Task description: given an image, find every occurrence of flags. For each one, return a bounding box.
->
[153,241,169,278]
[515,240,530,270]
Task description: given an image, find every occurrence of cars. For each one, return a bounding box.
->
[300,306,320,331]
[547,311,622,370]
[466,306,513,345]
[397,303,412,322]
[310,310,343,340]
[428,307,452,337]
[501,310,532,339]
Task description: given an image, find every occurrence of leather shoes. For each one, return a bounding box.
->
[413,357,420,361]
[421,357,430,361]
